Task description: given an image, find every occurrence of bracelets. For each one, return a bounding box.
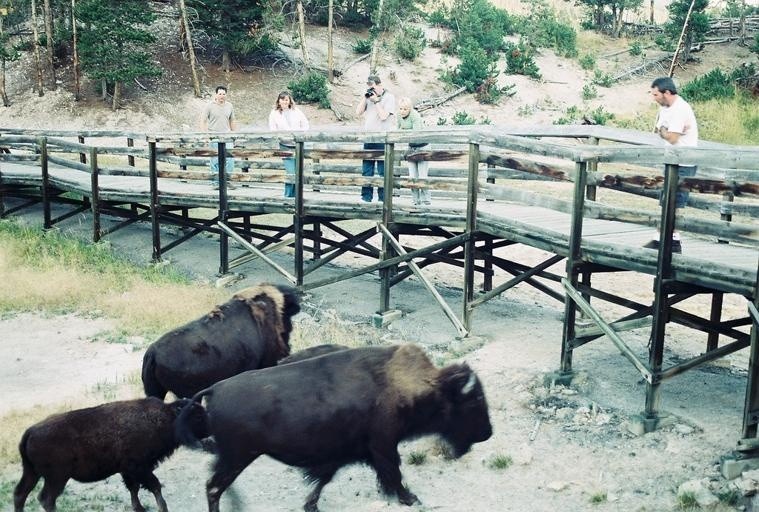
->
[659,126,668,132]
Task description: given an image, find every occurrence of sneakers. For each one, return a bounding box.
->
[644,239,680,253]
[212,183,236,189]
[413,201,430,206]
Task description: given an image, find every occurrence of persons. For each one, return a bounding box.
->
[396,97,434,204]
[355,75,396,206]
[202,86,239,191]
[645,76,700,255]
[268,91,310,197]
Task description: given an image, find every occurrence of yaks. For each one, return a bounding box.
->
[172,338,494,512]
[13,394,207,512]
[141,281,304,402]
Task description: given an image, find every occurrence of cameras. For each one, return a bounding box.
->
[365,87,377,99]
